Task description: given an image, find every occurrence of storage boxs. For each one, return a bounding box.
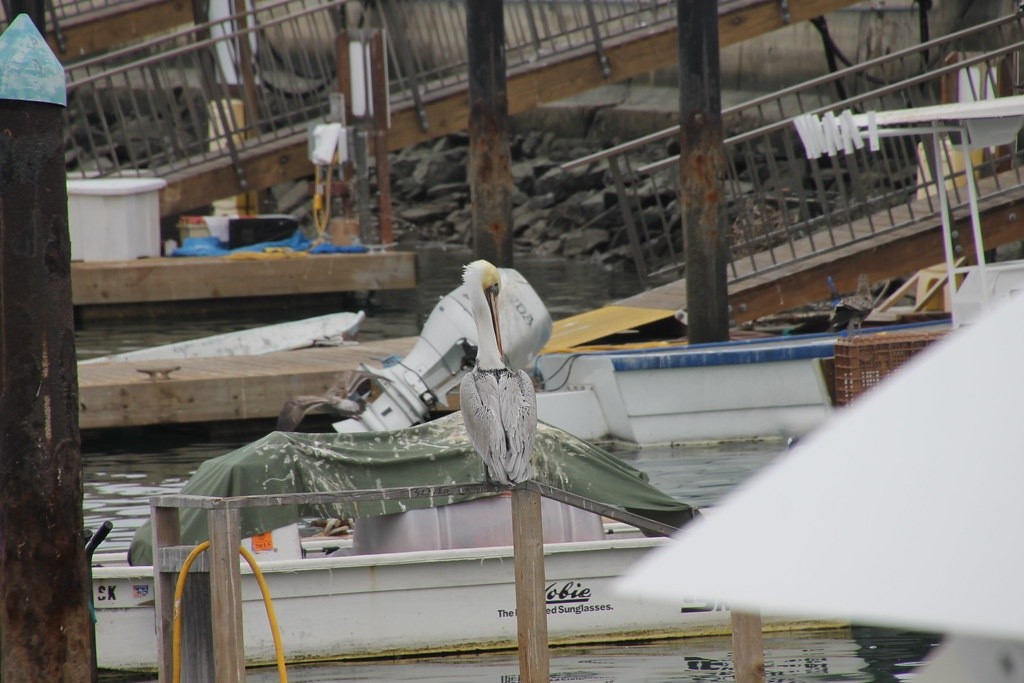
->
[352,491,607,556]
[68,175,301,260]
[834,327,952,406]
[240,520,301,565]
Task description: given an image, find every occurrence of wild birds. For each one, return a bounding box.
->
[458,260,537,487]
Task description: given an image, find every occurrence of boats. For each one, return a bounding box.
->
[536,317,952,444]
[178,212,298,246]
[76,308,369,365]
[88,260,860,671]
